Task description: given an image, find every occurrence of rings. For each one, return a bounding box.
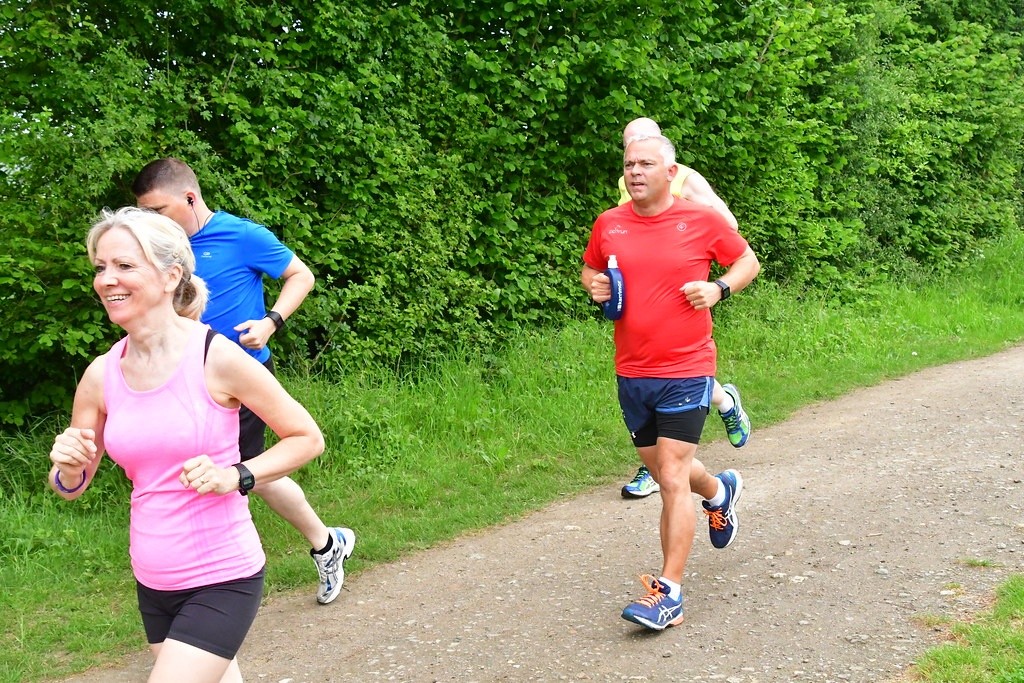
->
[199,475,208,484]
[693,300,697,306]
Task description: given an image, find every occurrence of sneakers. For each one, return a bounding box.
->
[310,526,355,604]
[718,384,751,447]
[702,469,743,548]
[621,574,684,630]
[622,463,661,497]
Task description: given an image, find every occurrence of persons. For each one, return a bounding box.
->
[619,118,752,497]
[132,158,357,603]
[48,207,328,683]
[581,131,744,630]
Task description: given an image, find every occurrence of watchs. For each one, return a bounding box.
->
[231,461,255,496]
[713,277,731,300]
[263,309,285,330]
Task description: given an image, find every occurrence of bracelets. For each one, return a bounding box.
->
[55,469,87,492]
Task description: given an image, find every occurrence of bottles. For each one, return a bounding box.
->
[602,254,623,320]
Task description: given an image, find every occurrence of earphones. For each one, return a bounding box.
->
[187,197,193,206]
